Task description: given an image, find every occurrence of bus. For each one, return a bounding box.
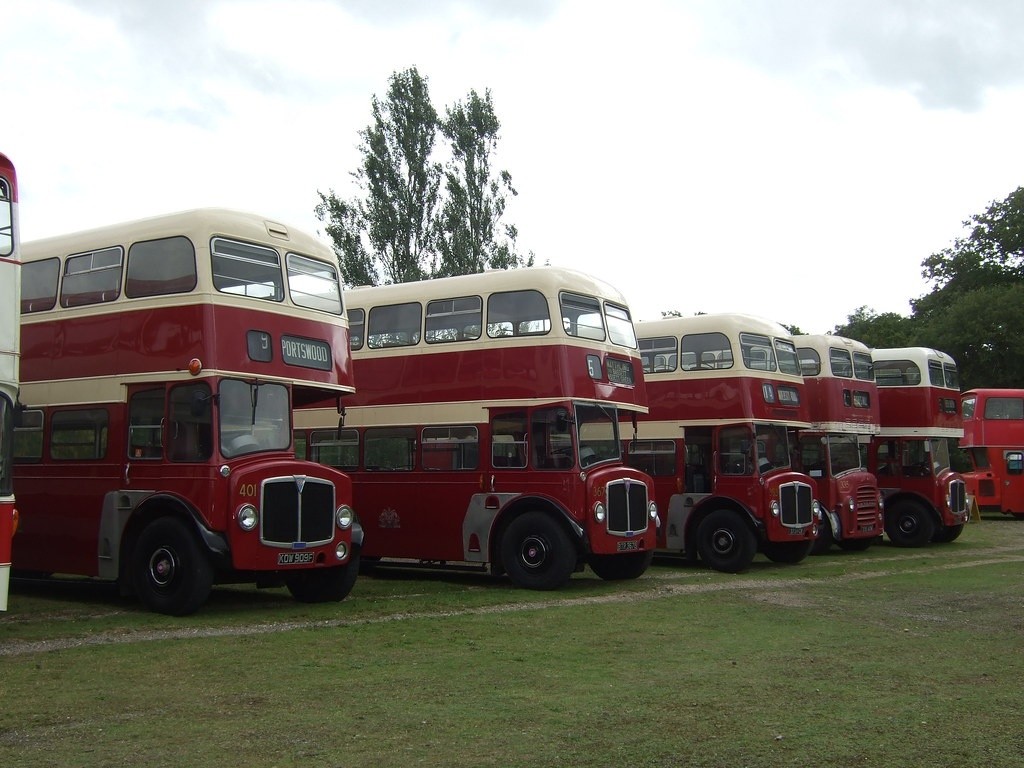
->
[17,208,371,608]
[260,268,660,588]
[640,345,969,549]
[597,336,889,551]
[416,313,822,560]
